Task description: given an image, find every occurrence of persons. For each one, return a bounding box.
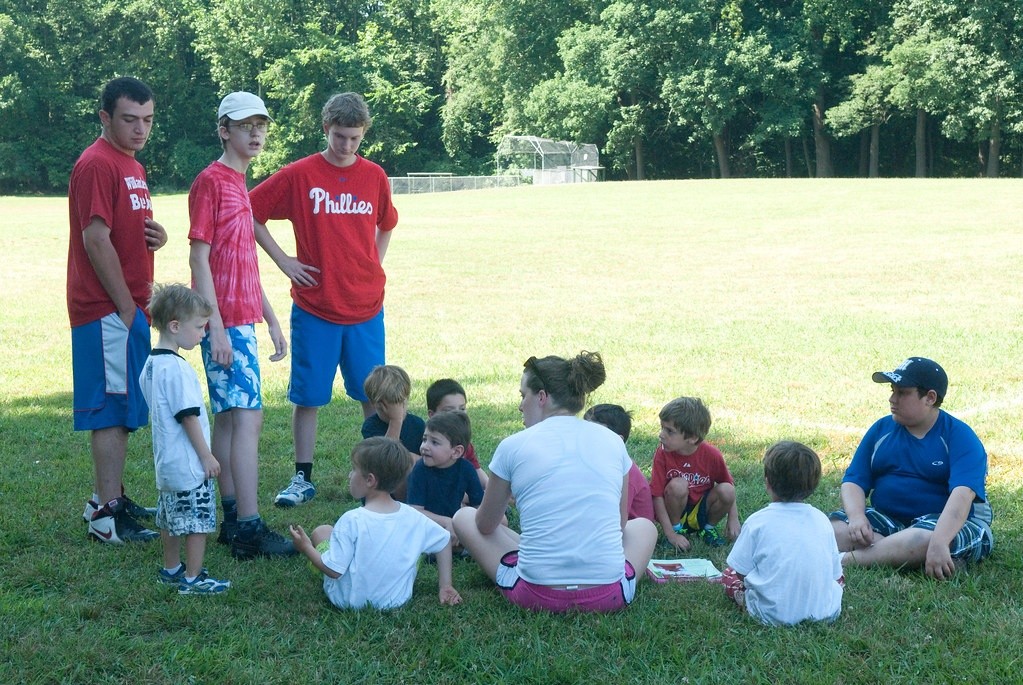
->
[828,357,994,580]
[188,91,299,561]
[139,285,233,594]
[427,379,515,527]
[404,408,484,547]
[649,397,741,555]
[66,77,168,547]
[584,404,631,443]
[289,436,463,612]
[361,365,426,505]
[723,440,843,627]
[247,92,398,506]
[452,352,658,613]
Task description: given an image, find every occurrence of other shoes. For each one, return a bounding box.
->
[460,549,469,558]
[426,555,436,564]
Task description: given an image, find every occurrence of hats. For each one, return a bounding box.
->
[218,91,274,122]
[872,357,947,398]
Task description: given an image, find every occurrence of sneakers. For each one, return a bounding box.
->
[231,523,301,561]
[698,528,728,548]
[82,482,157,523]
[274,470,316,507]
[662,528,688,548]
[177,567,233,595]
[87,497,160,546]
[217,521,237,544]
[156,562,210,586]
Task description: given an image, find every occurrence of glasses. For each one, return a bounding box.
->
[523,356,548,398]
[226,122,270,133]
